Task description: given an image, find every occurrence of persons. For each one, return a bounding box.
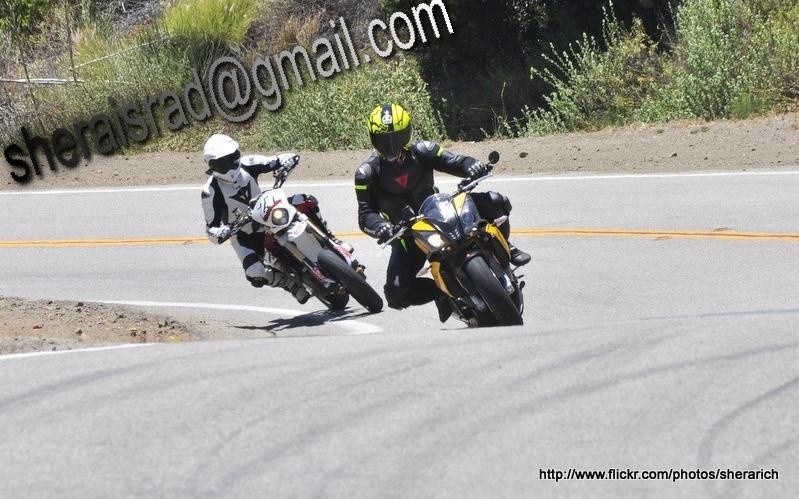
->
[353,102,530,324]
[198,132,355,305]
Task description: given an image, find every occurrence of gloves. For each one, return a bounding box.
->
[217,225,230,238]
[278,153,297,169]
[468,160,487,179]
[375,222,393,243]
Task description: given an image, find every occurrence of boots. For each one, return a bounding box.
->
[269,266,310,304]
[420,277,453,322]
[327,229,353,253]
[497,218,531,266]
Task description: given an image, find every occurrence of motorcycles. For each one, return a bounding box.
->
[217,153,384,315]
[375,151,526,326]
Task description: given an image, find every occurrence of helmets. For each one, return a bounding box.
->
[368,103,413,160]
[203,134,241,182]
